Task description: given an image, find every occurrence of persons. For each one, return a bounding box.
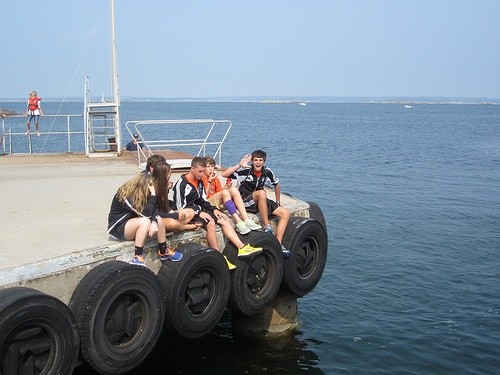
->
[173,156,263,270]
[106,155,201,266]
[125,134,144,151]
[25,91,43,136]
[222,151,291,257]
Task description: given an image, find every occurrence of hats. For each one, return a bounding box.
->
[133,134,138,138]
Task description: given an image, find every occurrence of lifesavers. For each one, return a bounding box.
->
[304,197,327,234]
[155,241,231,339]
[68,259,165,375]
[274,215,329,298]
[264,184,273,189]
[223,227,284,319]
[282,191,293,197]
[0,285,81,375]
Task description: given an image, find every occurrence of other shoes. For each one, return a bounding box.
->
[235,221,251,234]
[237,243,263,257]
[223,254,236,270]
[245,220,262,230]
[264,225,273,233]
[281,244,291,257]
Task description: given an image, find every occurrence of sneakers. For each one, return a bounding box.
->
[158,247,183,262]
[128,255,146,267]
